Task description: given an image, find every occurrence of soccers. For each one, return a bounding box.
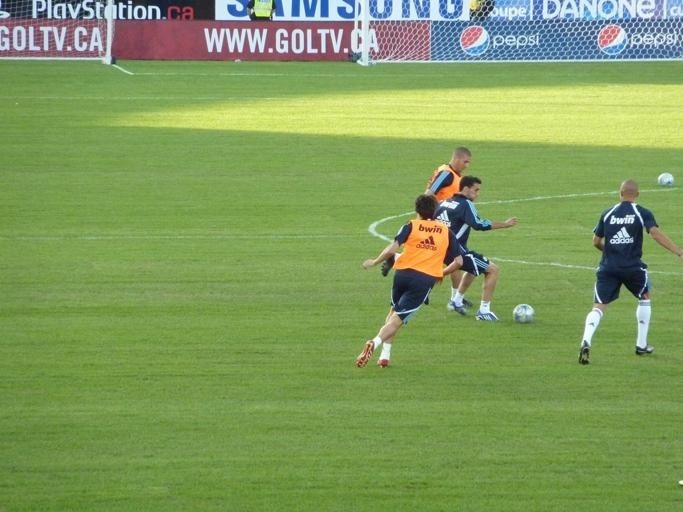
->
[658,171,675,188]
[513,304,535,324]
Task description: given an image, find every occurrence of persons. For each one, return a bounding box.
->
[578,180,683,364]
[432,176,518,321]
[356,195,464,368]
[381,147,473,307]
[247,0,276,21]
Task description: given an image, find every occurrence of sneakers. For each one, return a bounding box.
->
[381,253,396,278]
[447,297,474,316]
[635,345,654,355]
[354,339,375,368]
[475,309,499,321]
[377,358,390,369]
[577,340,591,366]
